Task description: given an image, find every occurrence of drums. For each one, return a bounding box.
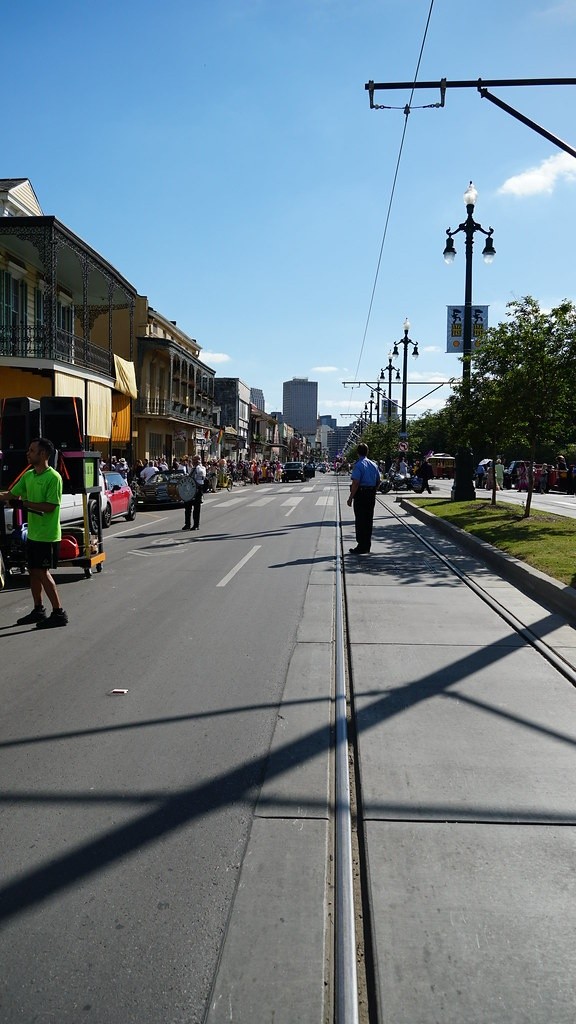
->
[167,473,197,502]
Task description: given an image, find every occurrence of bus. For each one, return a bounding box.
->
[427,453,455,480]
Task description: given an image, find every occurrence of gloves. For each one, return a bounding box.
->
[12,499,23,509]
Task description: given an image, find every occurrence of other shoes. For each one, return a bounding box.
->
[349,546,370,553]
[517,490,521,492]
[191,525,200,530]
[182,525,191,530]
[211,490,217,493]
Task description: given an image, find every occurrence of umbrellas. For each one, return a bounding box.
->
[479,459,493,465]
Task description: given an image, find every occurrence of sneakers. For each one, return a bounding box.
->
[17,608,47,625]
[36,611,68,628]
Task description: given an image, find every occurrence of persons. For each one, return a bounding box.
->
[103,455,282,496]
[0,438,68,628]
[322,459,340,475]
[182,455,207,531]
[476,458,576,494]
[347,443,380,553]
[389,457,433,495]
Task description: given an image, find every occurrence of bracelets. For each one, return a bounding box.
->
[349,496,353,499]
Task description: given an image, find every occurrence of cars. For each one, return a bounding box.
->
[281,462,306,483]
[102,472,137,529]
[136,470,188,507]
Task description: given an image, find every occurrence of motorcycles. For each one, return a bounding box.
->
[379,463,423,494]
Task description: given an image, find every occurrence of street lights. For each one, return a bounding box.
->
[378,348,401,479]
[442,179,496,503]
[355,390,379,436]
[391,318,419,461]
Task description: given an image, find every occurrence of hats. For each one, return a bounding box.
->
[119,458,127,464]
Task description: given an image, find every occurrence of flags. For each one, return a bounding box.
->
[204,431,208,439]
[216,431,223,445]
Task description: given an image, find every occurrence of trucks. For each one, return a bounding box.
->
[0,466,107,537]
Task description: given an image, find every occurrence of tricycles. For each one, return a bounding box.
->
[206,473,233,492]
